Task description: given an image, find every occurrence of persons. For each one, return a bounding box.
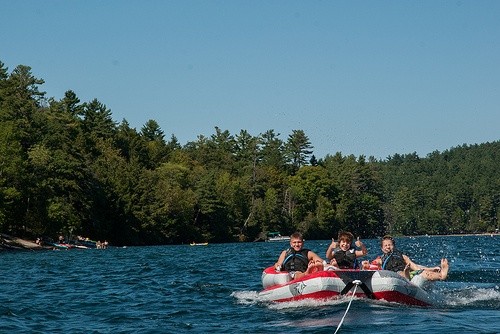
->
[362,236,441,279]
[96,240,108,249]
[59,235,64,243]
[77,234,89,241]
[326,232,367,269]
[36,238,40,245]
[274,233,324,280]
[410,257,448,291]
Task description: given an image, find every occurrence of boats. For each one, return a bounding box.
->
[190,243,209,246]
[258,265,436,309]
[264,232,305,242]
